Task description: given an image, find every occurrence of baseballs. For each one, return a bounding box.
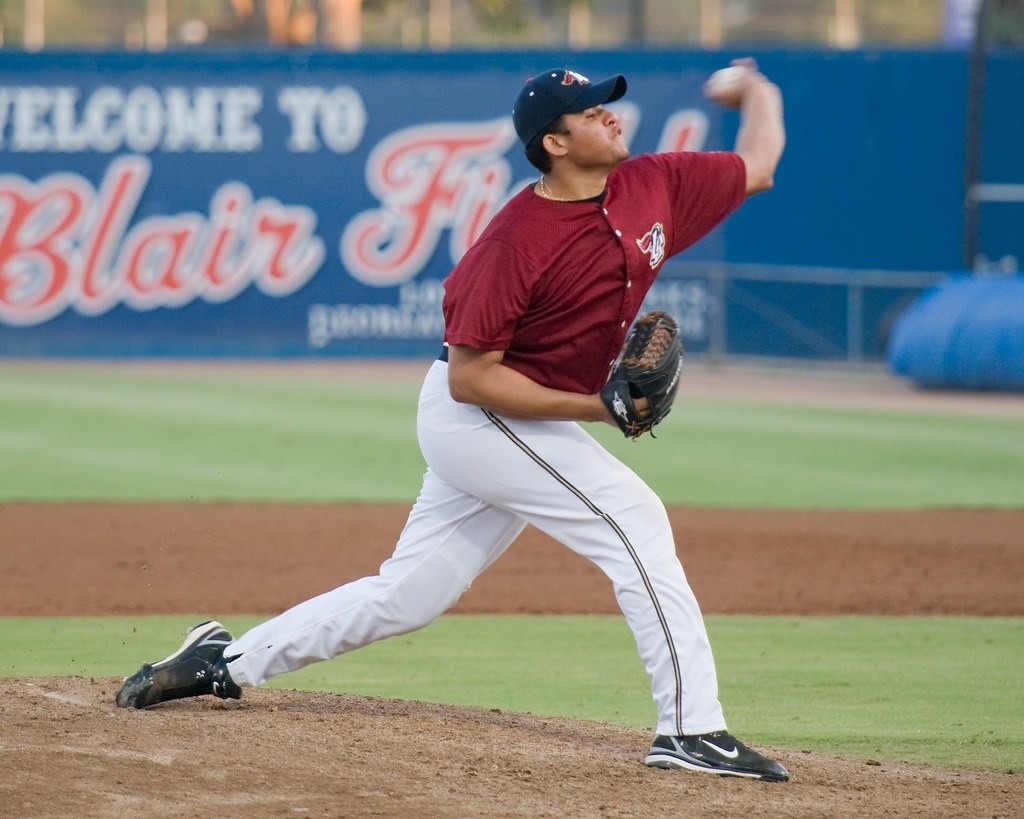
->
[707,65,747,91]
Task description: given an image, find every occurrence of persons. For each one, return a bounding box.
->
[113,65,788,783]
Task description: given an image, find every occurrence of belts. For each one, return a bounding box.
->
[437,345,449,362]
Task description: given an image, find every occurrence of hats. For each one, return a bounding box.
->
[512,69,627,149]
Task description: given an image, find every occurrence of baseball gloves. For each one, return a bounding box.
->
[599,310,686,439]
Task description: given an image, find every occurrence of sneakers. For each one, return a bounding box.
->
[645,730,790,784]
[116,620,242,707]
